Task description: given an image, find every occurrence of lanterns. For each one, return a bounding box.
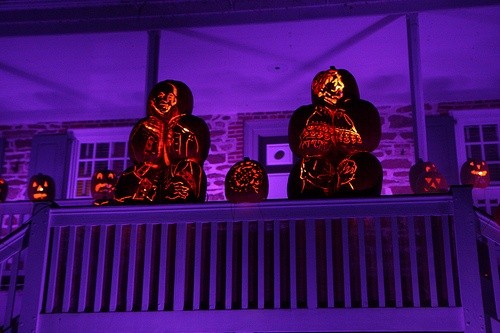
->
[92,169,118,199]
[0,179,8,201]
[460,158,491,187]
[28,173,55,202]
[408,161,446,193]
